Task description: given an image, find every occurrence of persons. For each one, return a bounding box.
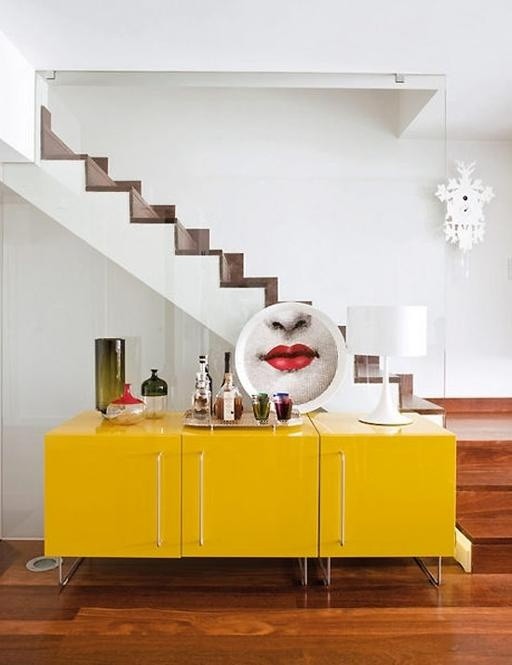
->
[242,308,338,405]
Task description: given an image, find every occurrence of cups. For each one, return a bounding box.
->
[273,393,292,424]
[252,392,270,424]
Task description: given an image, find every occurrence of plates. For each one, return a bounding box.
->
[235,301,347,415]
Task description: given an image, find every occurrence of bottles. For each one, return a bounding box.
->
[142,368,167,419]
[107,383,145,425]
[213,352,243,421]
[192,356,212,424]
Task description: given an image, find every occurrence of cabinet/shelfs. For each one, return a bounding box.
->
[45,412,456,591]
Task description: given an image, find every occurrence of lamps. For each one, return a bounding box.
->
[345,303,428,425]
